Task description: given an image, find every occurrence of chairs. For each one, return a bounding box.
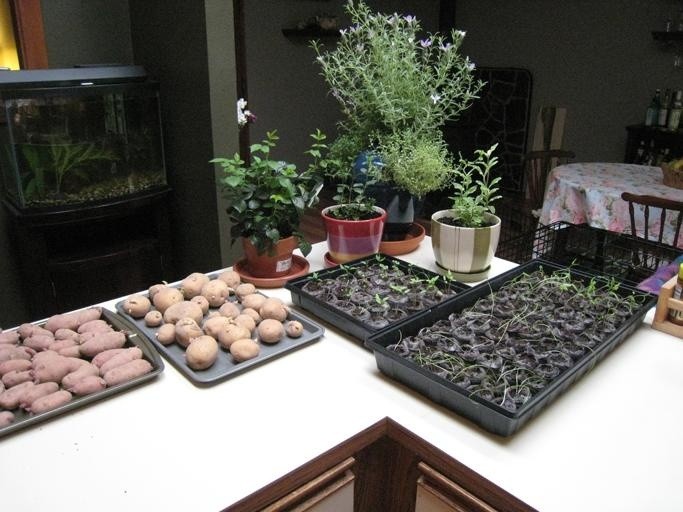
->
[621,192,683,270]
[515,151,576,261]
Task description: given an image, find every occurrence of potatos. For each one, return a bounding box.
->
[122,269,303,371]
[0,305,155,429]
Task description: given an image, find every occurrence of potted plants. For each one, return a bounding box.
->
[430,142,501,273]
[321,155,386,264]
[209,129,327,278]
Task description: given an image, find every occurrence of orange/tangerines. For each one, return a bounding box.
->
[668,159,683,172]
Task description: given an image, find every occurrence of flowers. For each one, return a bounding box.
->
[307,0,488,201]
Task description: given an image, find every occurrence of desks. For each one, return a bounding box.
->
[0,235,682,512]
[0,187,172,321]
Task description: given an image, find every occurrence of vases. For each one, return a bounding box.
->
[366,186,414,240]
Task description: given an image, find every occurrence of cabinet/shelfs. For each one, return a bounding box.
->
[624,122,683,163]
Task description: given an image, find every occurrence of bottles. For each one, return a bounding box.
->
[671,264,683,325]
[638,87,683,167]
